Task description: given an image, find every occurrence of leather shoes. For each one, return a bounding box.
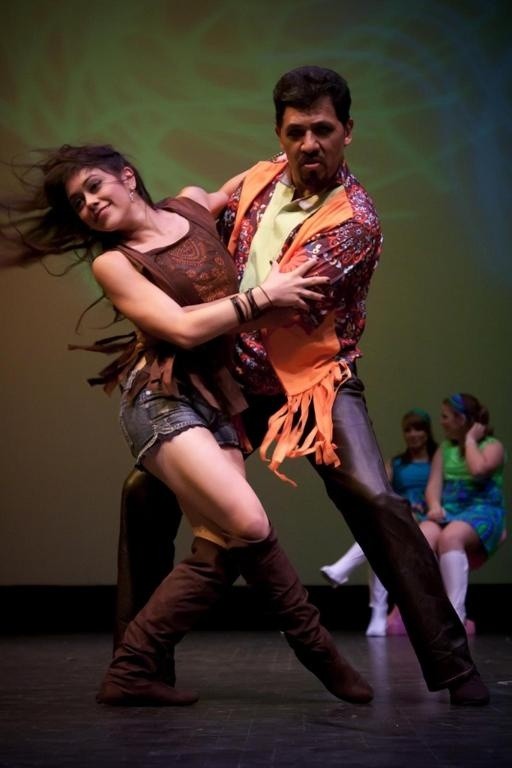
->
[444,671,491,706]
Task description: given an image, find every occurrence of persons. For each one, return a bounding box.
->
[320,410,439,635]
[386,392,506,635]
[115,65,490,704]
[1,145,376,705]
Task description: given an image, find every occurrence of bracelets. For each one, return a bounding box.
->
[230,286,273,325]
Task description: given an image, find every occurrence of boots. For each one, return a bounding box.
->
[98,537,243,704]
[439,551,468,625]
[224,522,372,704]
[365,573,388,636]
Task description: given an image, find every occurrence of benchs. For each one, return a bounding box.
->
[469,531,505,626]
